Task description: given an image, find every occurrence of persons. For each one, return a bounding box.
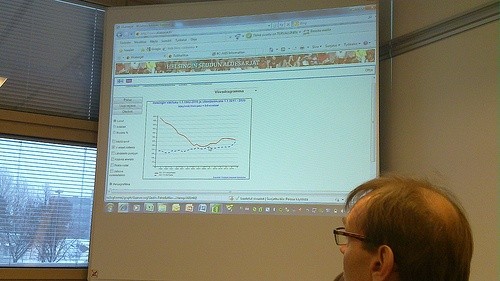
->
[339,171,474,281]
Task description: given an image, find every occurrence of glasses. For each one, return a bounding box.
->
[333,227,400,272]
